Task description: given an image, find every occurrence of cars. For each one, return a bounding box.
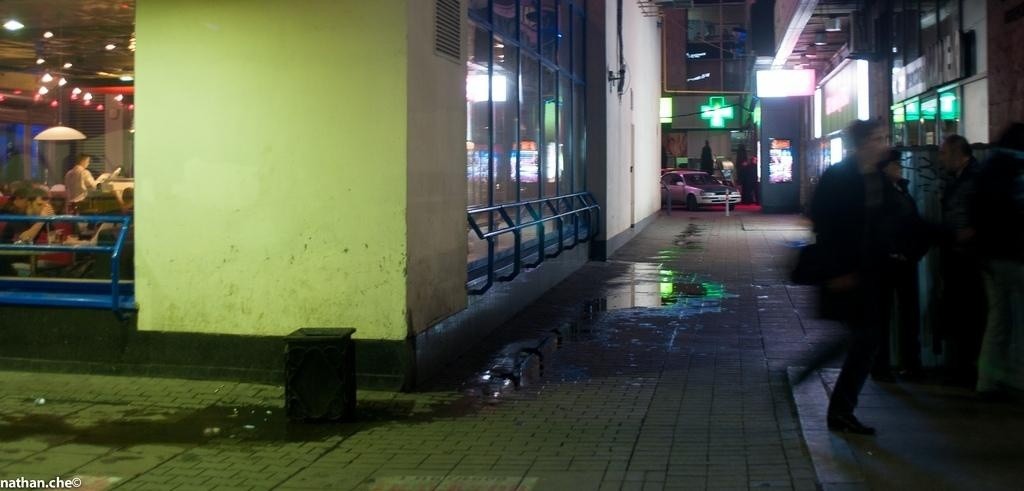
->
[661,170,743,211]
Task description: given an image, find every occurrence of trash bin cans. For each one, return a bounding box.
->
[280,328,356,424]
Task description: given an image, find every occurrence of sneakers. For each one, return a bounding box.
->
[872,369,926,384]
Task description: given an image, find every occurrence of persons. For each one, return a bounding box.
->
[874,150,927,382]
[694,23,747,59]
[978,122,1024,396]
[740,156,760,204]
[702,140,712,169]
[661,138,676,167]
[937,135,981,206]
[767,119,891,435]
[0,153,109,280]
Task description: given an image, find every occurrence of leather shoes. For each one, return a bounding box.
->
[827,411,876,435]
